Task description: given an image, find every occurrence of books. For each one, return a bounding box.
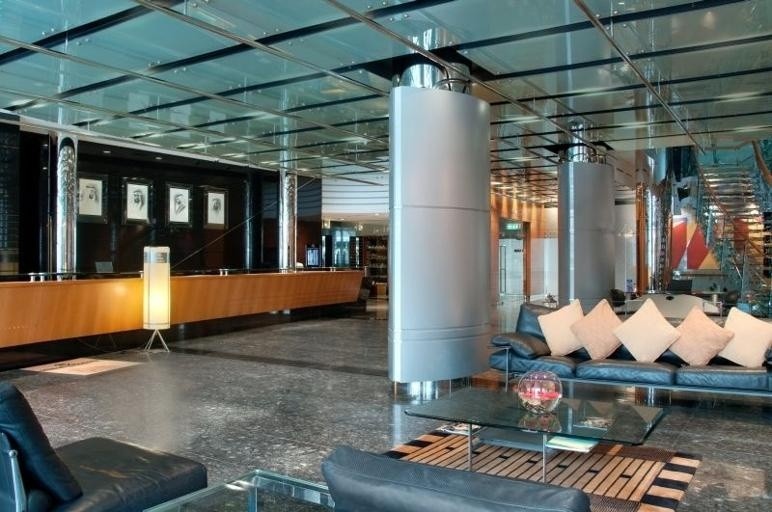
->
[546,435,600,454]
[435,420,489,437]
[573,416,616,432]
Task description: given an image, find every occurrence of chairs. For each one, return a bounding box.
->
[0,430,208,512]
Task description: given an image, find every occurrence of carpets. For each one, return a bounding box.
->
[381,421,704,511]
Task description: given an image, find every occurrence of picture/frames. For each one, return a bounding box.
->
[76,171,230,231]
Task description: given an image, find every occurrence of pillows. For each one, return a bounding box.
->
[490,298,772,369]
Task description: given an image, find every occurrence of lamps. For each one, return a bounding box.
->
[142,246,172,352]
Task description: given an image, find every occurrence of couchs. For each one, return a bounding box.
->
[488,302,771,407]
